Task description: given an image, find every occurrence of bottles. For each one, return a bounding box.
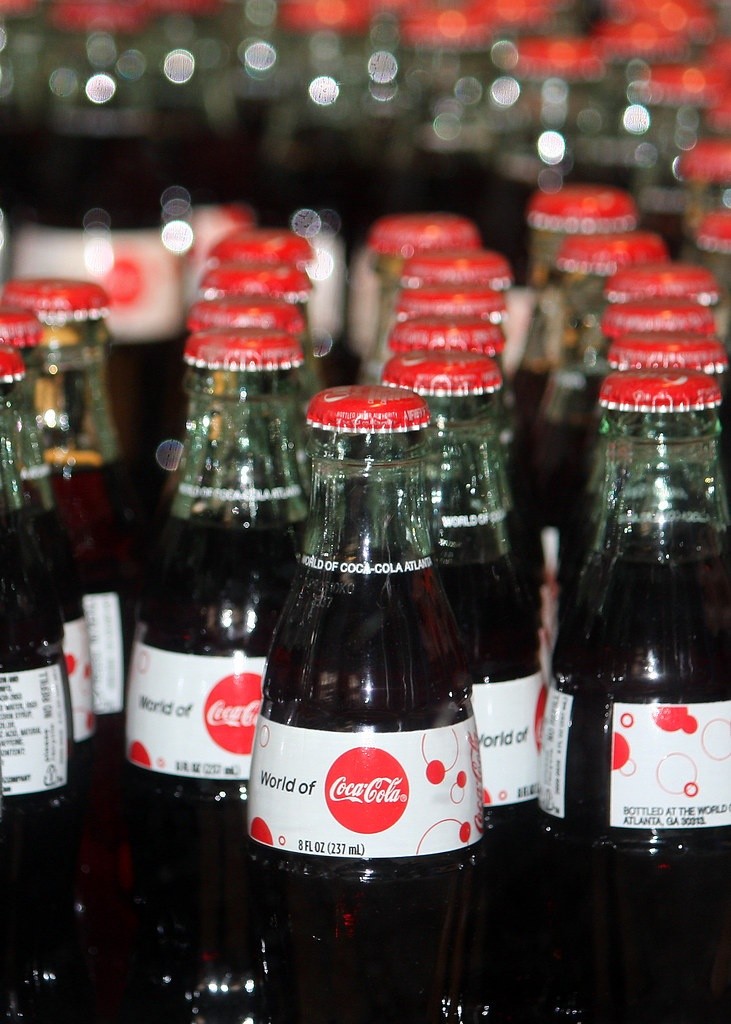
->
[0,0,731,1024]
